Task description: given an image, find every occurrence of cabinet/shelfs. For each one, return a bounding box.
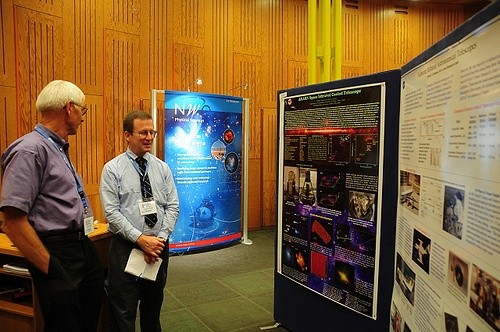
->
[0,222,114,332]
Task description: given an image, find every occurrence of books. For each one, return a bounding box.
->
[125,248,163,282]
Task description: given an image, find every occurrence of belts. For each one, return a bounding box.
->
[46,231,87,241]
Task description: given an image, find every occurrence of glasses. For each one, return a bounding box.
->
[132,130,157,138]
[63,102,88,116]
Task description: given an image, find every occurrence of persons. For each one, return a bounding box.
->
[99,109,180,332]
[0,80,105,332]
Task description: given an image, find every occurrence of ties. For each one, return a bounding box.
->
[135,157,157,228]
